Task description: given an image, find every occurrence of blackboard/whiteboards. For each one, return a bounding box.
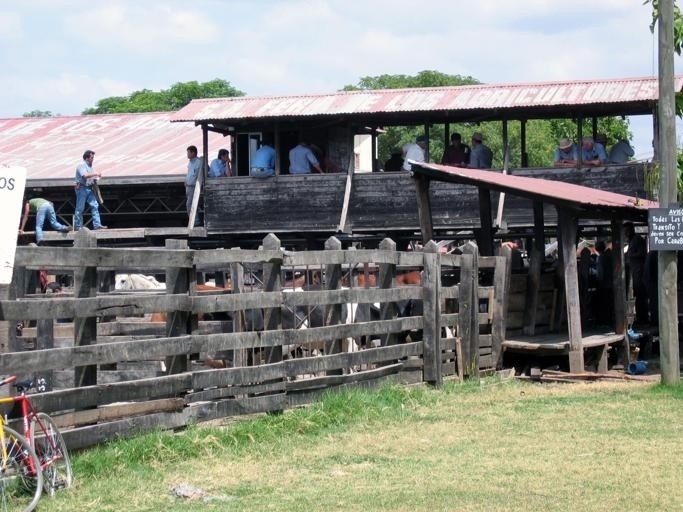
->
[648,207,683,251]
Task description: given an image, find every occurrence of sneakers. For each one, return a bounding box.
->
[95,225,108,229]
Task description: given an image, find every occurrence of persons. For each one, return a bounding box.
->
[466,131,493,169]
[184,146,205,226]
[401,133,428,173]
[581,137,607,166]
[209,149,232,177]
[440,132,470,169]
[553,138,580,168]
[578,223,682,355]
[605,138,635,164]
[19,197,73,246]
[250,139,276,179]
[288,133,325,175]
[384,145,406,173]
[75,150,108,231]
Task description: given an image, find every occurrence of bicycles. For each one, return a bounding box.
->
[0,374,73,511]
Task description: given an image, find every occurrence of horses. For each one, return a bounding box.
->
[114,270,424,389]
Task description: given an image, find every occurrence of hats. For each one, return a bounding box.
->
[559,138,573,149]
[416,135,430,144]
[595,134,609,143]
[470,132,487,140]
